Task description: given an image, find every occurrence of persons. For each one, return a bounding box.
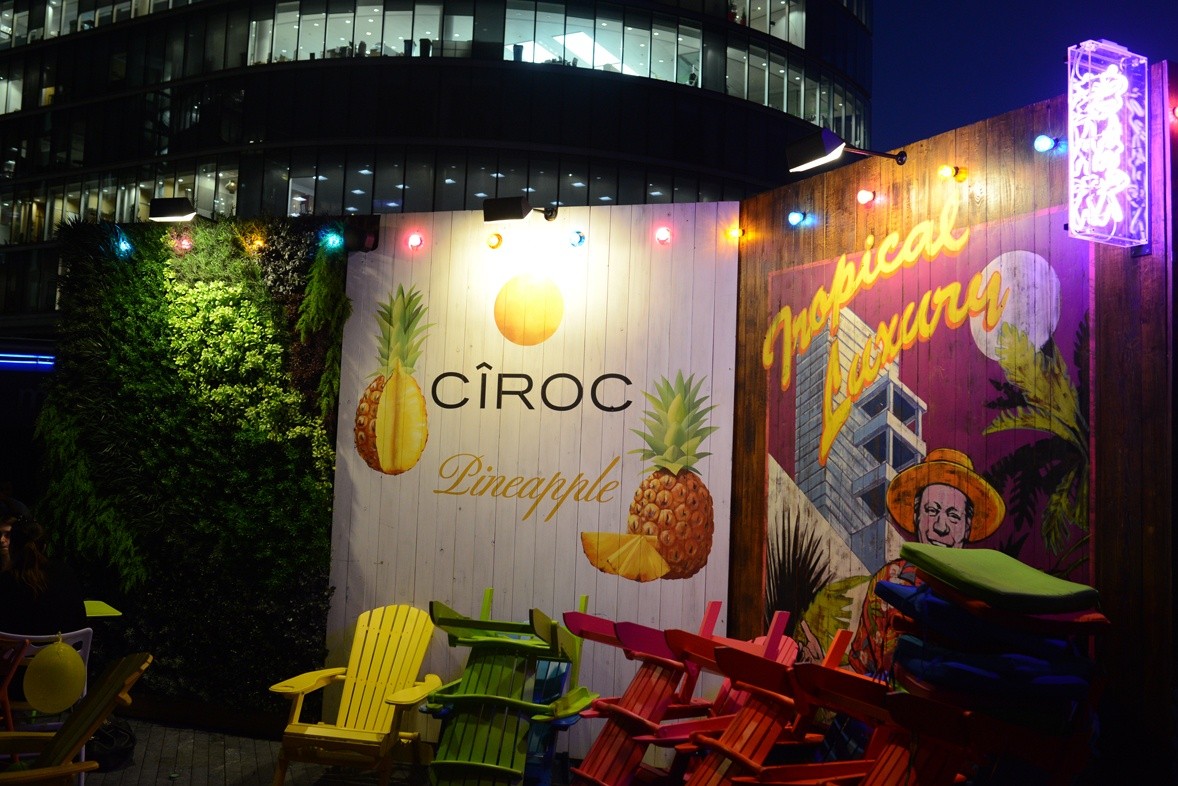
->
[0,495,81,726]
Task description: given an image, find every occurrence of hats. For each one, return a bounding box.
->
[0,496,37,524]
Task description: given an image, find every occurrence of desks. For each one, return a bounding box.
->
[84,600,122,618]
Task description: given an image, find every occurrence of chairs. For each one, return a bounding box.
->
[0,626,93,786]
[0,638,31,763]
[0,651,153,786]
[266,587,1106,786]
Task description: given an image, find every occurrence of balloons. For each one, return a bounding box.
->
[23,642,85,713]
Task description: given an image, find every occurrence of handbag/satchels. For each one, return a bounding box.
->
[75,717,137,770]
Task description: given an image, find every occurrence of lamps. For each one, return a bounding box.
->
[292,195,309,201]
[784,128,908,174]
[482,196,559,223]
[149,196,218,224]
[1066,36,1152,259]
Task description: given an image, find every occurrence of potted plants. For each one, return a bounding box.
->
[727,0,737,21]
[740,7,746,26]
